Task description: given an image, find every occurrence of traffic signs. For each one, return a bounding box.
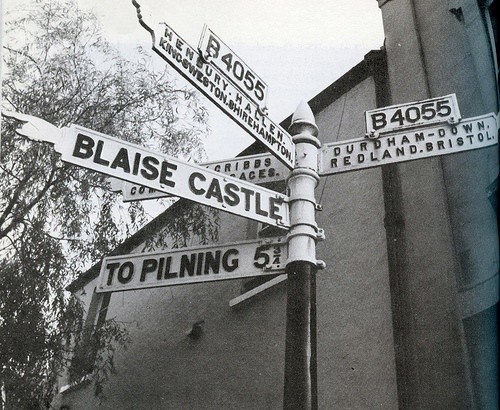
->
[1,0,500,293]
